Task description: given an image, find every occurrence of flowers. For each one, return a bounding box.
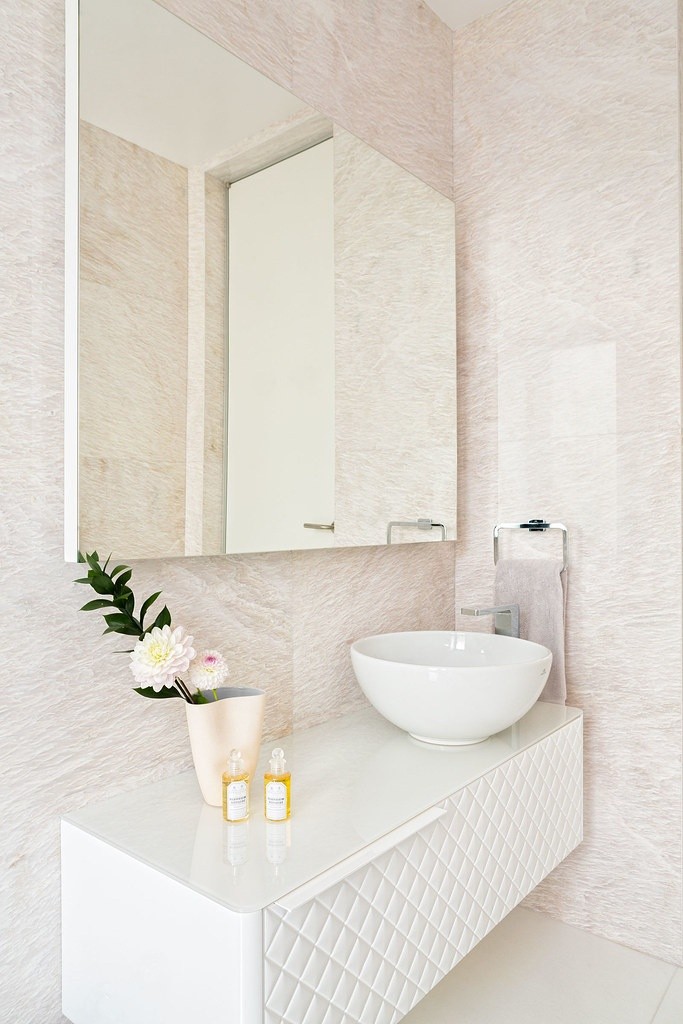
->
[74,551,231,706]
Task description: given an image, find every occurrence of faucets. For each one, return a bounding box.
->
[461,603,518,636]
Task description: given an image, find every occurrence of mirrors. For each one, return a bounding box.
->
[65,0,458,564]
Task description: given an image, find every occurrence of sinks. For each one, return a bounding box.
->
[349,630,552,746]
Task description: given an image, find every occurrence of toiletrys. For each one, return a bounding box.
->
[222,748,251,823]
[263,747,292,823]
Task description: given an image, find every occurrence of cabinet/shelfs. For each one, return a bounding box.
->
[58,716,586,1024]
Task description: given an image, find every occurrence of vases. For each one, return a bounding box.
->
[180,690,265,811]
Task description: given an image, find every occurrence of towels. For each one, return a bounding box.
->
[494,557,567,706]
